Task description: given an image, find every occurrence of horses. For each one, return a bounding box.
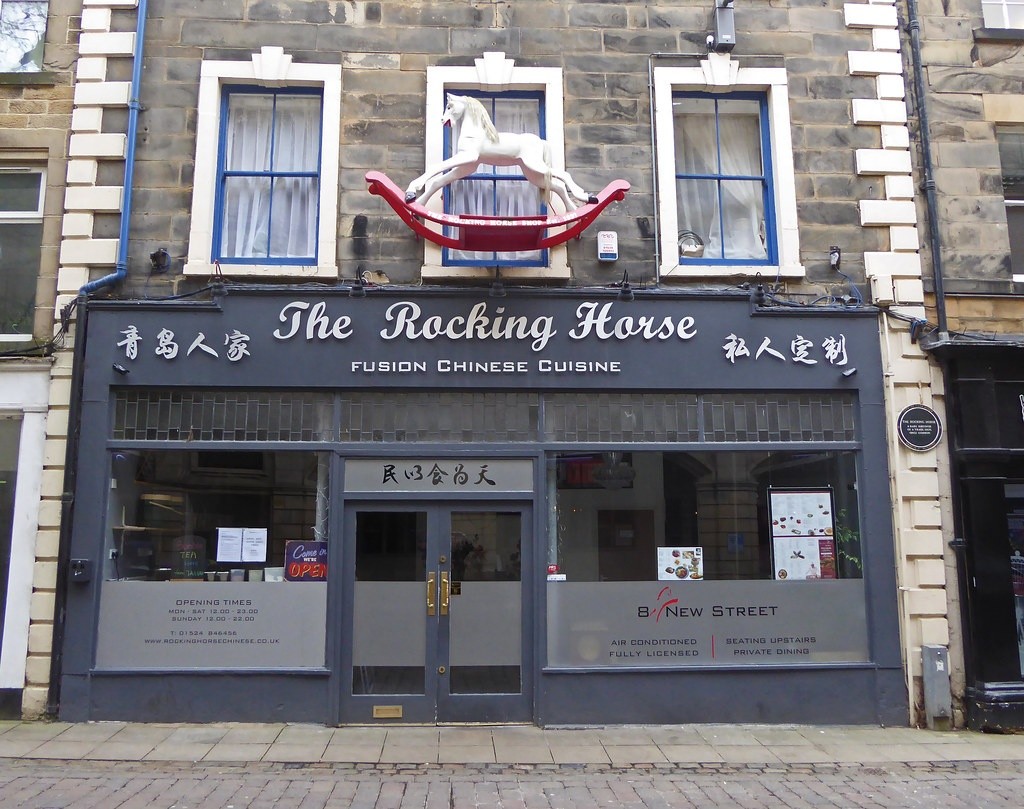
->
[404,93,598,240]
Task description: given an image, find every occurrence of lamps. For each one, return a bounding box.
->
[348,265,366,298]
[210,263,229,296]
[488,264,507,297]
[618,269,634,302]
[742,271,767,304]
